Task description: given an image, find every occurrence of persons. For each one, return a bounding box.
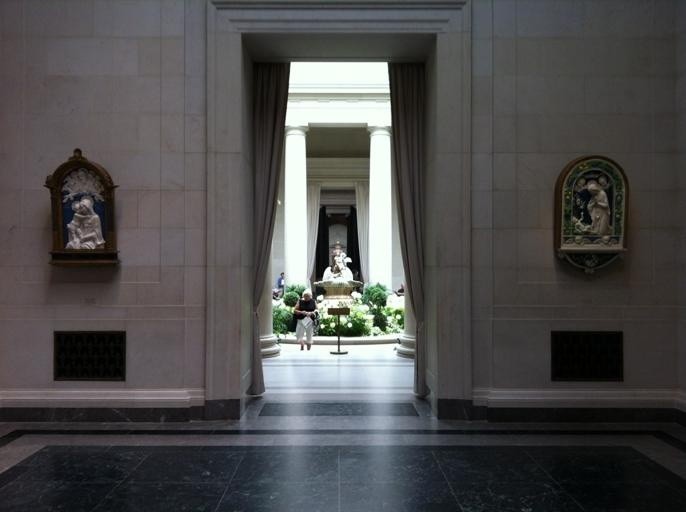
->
[66,201,89,243]
[587,185,610,234]
[67,197,105,249]
[572,212,599,234]
[294,289,319,350]
[277,273,284,296]
